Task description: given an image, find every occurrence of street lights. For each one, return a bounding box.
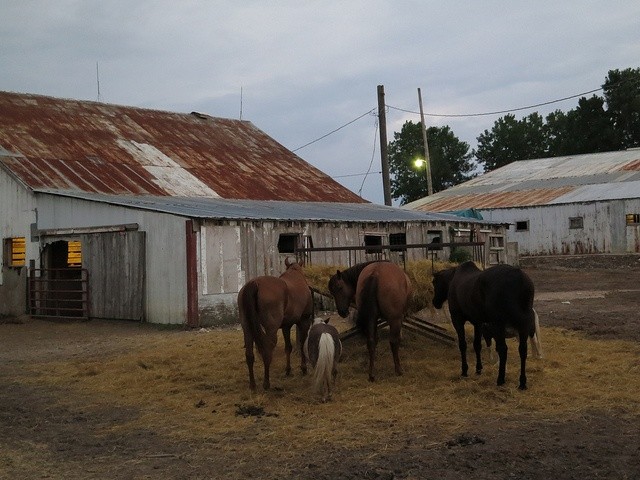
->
[414,159,433,196]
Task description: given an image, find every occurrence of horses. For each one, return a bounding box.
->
[328,260,413,383]
[303,317,342,402]
[482,307,543,359]
[237,255,314,395]
[431,261,535,390]
